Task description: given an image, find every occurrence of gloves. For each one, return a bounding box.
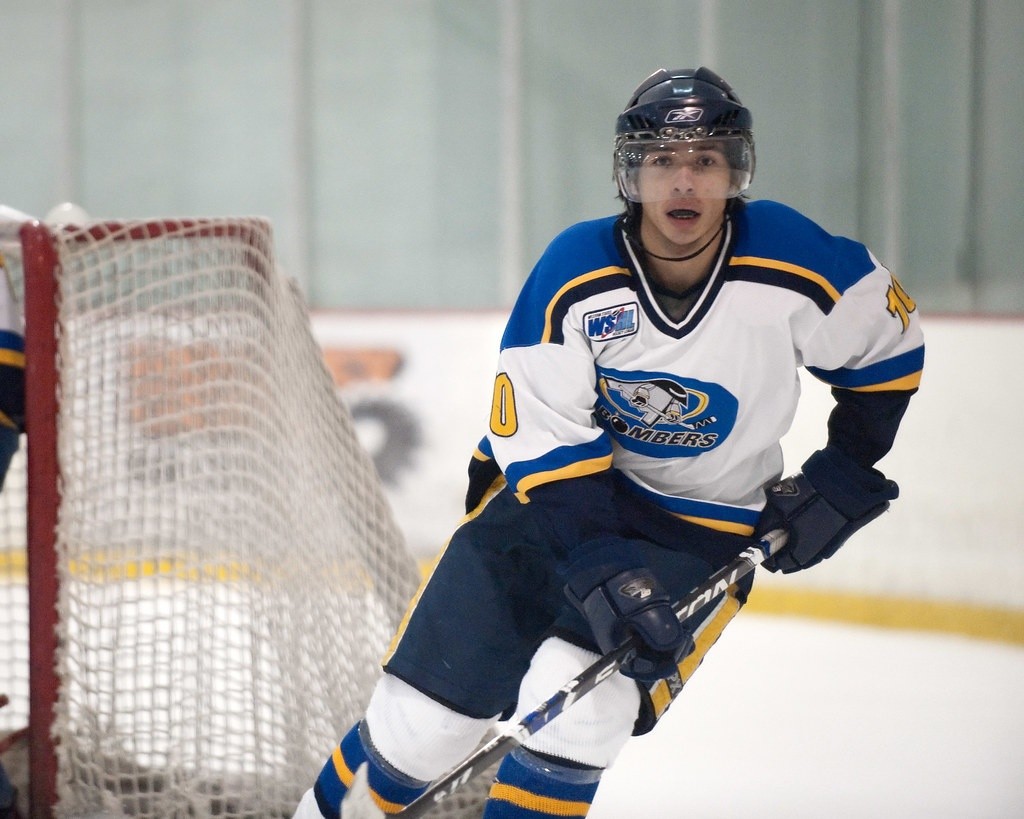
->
[751,446,899,574]
[563,555,696,683]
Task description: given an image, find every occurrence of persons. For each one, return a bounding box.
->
[286,67,926,819]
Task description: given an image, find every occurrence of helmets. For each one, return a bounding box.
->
[614,67,756,185]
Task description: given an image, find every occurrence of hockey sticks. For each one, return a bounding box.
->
[385,529,792,819]
